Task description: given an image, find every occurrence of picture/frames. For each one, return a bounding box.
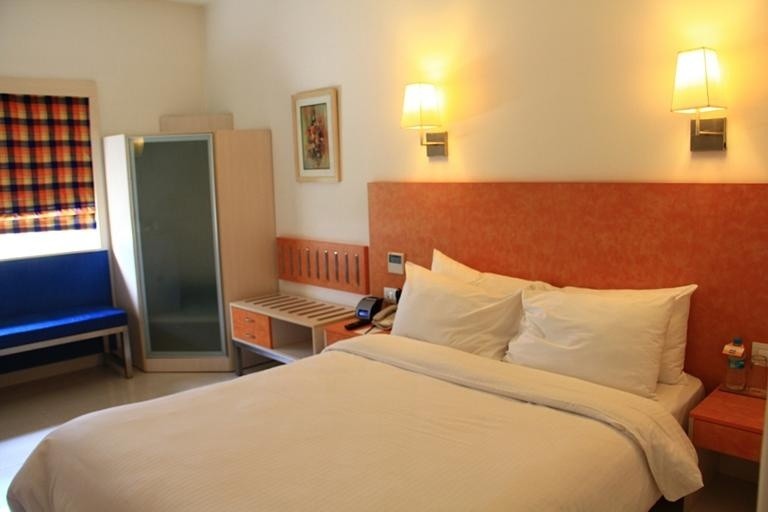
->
[289,86,341,183]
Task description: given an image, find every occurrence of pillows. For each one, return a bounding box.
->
[559,283,698,384]
[390,260,524,360]
[502,287,680,400]
[432,245,552,287]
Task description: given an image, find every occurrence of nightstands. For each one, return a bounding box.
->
[685,381,766,512]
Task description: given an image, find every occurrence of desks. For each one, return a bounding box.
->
[229,291,356,366]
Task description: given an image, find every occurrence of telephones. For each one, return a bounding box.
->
[371,305,397,330]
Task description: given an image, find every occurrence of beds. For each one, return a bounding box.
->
[31,177,766,511]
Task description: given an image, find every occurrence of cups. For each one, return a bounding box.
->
[749,354,768,397]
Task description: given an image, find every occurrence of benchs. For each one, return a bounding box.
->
[2,250,135,381]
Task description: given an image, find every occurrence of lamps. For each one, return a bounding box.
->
[398,84,449,161]
[669,44,732,154]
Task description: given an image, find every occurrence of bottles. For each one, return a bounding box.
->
[726,338,747,391]
